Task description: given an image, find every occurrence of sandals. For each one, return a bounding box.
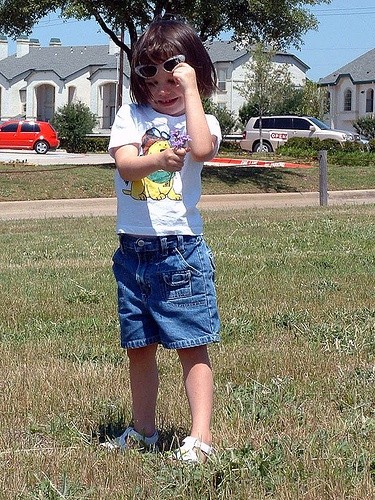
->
[166,436,214,467]
[99,427,160,453]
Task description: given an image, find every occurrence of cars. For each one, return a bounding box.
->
[0,120,60,155]
[239,116,362,153]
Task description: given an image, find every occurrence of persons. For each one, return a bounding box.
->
[96,19,223,469]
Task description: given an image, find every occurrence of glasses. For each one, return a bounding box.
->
[134,54,185,79]
